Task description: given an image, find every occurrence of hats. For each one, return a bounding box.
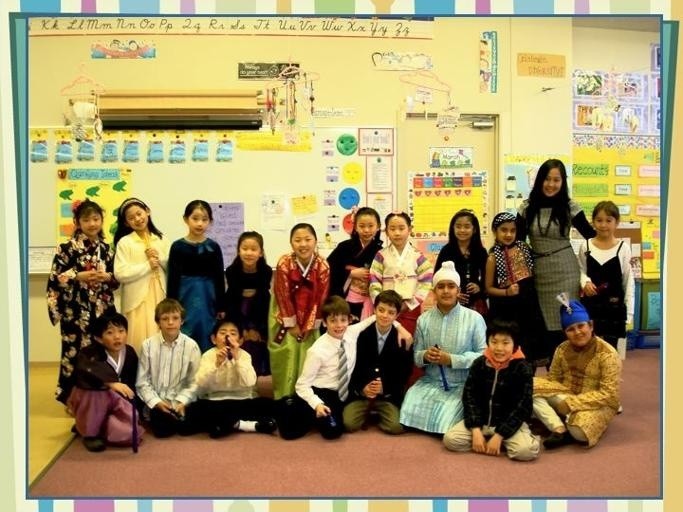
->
[432,260,461,292]
[556,292,590,329]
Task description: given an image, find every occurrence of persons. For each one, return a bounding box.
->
[131,298,204,439]
[65,313,143,452]
[164,199,225,350]
[44,199,119,416]
[112,199,170,361]
[228,156,637,463]
[191,320,277,442]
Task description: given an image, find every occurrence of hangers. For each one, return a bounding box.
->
[401,68,452,94]
[58,61,107,97]
[263,53,321,90]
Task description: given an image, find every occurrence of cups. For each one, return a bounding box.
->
[413,176,482,188]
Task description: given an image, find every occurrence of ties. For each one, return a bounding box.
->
[337,341,349,402]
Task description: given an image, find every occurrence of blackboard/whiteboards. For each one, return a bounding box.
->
[28,124,397,276]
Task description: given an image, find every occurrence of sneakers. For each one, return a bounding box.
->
[82,437,105,451]
[543,431,575,448]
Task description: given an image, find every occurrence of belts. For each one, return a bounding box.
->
[531,245,571,258]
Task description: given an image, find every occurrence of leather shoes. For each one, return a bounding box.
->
[250,417,276,433]
